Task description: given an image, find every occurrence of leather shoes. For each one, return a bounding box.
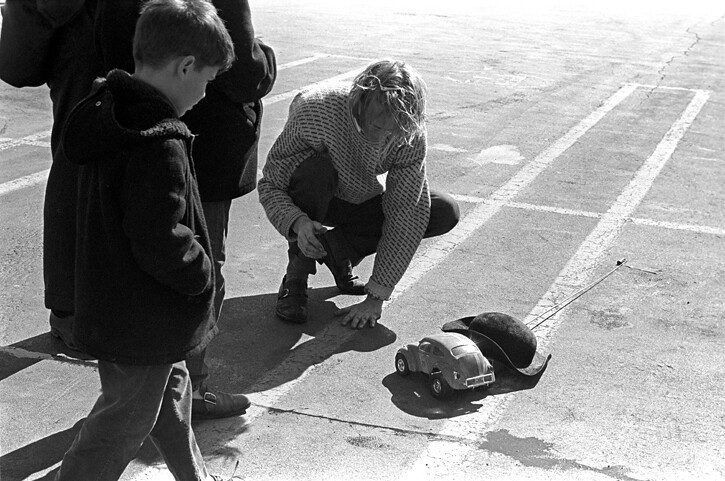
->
[191,383,251,419]
[275,274,308,323]
[316,231,366,295]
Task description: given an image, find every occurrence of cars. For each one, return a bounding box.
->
[395,332,496,401]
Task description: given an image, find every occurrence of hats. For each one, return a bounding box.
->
[442,313,552,376]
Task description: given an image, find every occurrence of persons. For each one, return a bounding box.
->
[0,0,275,419]
[43,0,234,481]
[255,57,460,328]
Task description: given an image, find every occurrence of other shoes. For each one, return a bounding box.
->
[49,310,77,351]
